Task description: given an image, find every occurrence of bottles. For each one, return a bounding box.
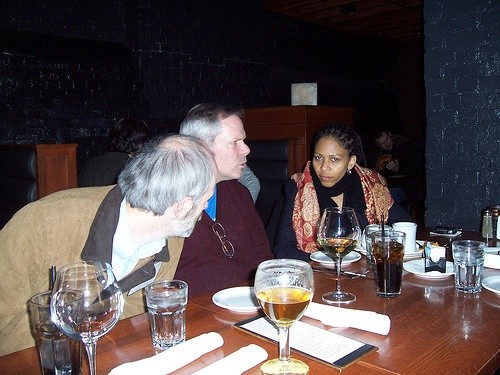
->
[481,209,496,247]
[493,208,500,242]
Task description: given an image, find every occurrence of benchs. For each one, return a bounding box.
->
[239,137,310,223]
[0,143,79,229]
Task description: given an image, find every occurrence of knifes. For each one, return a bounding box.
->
[313,265,373,279]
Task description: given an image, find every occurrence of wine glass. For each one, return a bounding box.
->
[254,259,314,375]
[50,260,125,375]
[316,207,362,304]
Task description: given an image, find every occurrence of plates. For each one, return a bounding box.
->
[481,276,500,294]
[212,286,261,313]
[402,259,455,278]
[310,250,362,266]
[356,240,432,259]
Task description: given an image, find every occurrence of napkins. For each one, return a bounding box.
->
[192,344,268,375]
[108,331,225,375]
[303,302,390,336]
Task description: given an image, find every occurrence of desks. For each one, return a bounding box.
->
[0,226,500,375]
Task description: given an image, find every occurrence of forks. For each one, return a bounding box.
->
[311,264,370,279]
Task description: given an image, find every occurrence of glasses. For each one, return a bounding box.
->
[212,223,235,258]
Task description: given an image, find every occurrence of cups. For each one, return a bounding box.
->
[371,230,406,298]
[394,222,417,260]
[365,224,392,259]
[452,240,485,294]
[145,279,189,351]
[30,289,84,375]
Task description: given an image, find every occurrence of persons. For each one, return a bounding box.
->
[273,122,415,263]
[374,128,409,203]
[0,130,220,358]
[170,99,274,300]
[76,116,152,188]
